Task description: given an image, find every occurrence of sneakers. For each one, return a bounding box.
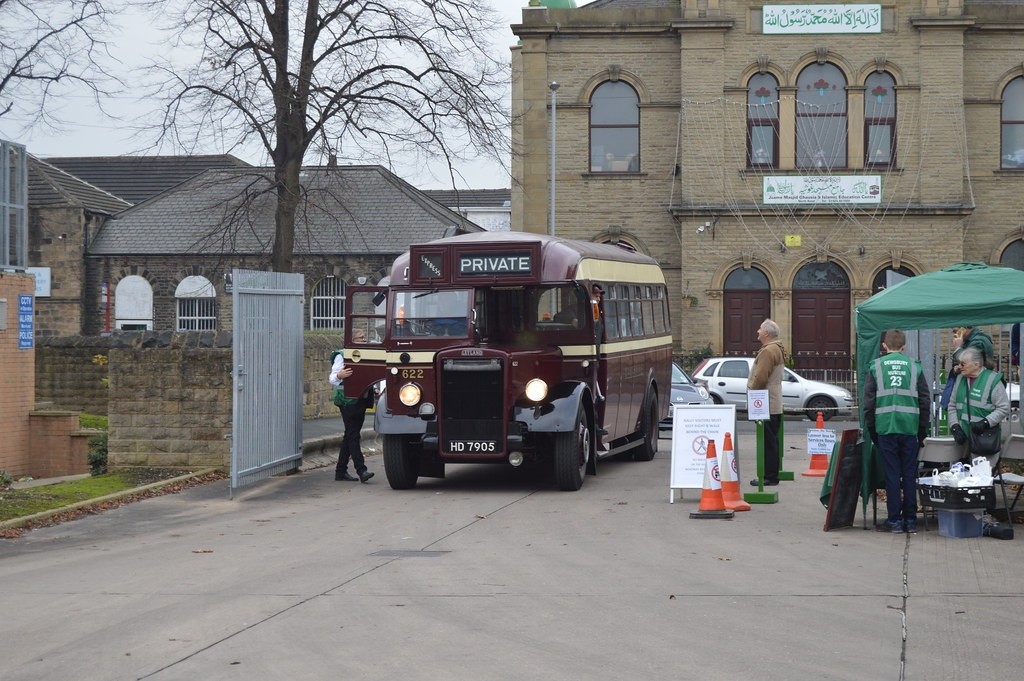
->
[904,520,917,532]
[876,519,903,532]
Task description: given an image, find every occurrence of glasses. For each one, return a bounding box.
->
[354,335,365,340]
[959,362,969,366]
[952,329,958,334]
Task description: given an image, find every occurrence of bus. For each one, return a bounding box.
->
[343,230,674,492]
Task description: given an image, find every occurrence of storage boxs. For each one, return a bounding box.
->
[915,484,997,510]
[936,507,986,538]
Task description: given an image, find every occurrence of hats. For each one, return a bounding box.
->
[592,285,605,294]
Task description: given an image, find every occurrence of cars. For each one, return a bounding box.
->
[937,380,1021,423]
[659,360,715,431]
[692,357,855,422]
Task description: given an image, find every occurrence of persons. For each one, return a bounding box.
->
[862,329,930,534]
[952,325,993,362]
[554,285,605,344]
[948,348,1010,510]
[329,328,375,482]
[747,319,785,487]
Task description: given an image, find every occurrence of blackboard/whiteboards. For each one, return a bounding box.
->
[824,428,863,532]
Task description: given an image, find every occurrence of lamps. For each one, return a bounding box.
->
[697,226,704,232]
[58,234,66,239]
[859,245,864,254]
[781,242,786,252]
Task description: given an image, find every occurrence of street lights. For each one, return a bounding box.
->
[550,81,562,237]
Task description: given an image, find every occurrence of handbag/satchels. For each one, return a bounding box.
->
[969,426,1002,456]
[1012,355,1017,365]
[920,457,994,503]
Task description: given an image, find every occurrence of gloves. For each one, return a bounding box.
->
[950,424,967,445]
[870,433,879,448]
[918,432,928,447]
[972,419,990,435]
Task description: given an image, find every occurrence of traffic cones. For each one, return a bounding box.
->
[802,411,830,476]
[720,432,751,510]
[690,439,736,519]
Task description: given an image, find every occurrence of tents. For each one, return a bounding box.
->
[855,261,1024,528]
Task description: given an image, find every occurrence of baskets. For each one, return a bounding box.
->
[918,484,993,508]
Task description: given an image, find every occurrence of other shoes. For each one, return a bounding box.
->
[594,424,608,435]
[750,478,779,485]
[359,468,374,482]
[335,472,358,480]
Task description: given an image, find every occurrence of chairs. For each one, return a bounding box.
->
[900,437,970,530]
[993,434,1024,527]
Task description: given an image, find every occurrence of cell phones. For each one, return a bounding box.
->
[957,327,966,337]
